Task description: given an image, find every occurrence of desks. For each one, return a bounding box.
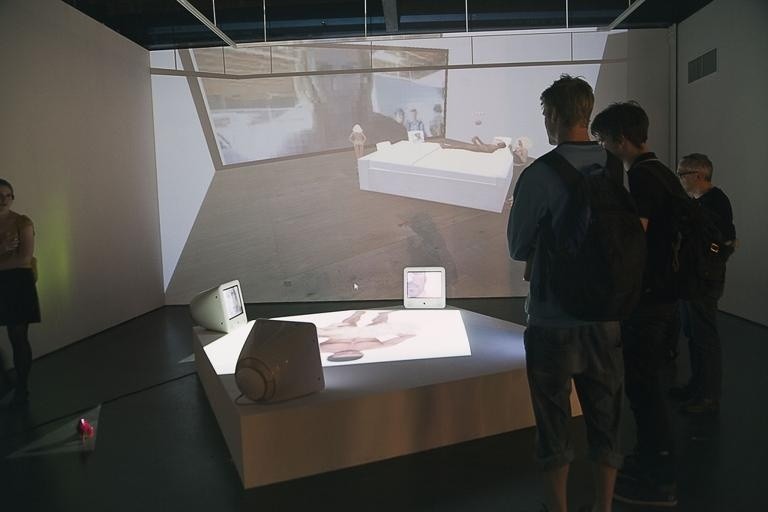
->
[193,302,584,489]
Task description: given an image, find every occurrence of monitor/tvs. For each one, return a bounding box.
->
[189,279,248,333]
[235,318,325,404]
[404,267,446,309]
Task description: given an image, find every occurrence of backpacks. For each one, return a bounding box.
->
[626,163,720,301]
[31,256,38,283]
[538,147,643,322]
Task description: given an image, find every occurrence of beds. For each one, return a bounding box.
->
[357,137,514,214]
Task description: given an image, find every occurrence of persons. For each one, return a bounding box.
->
[0,175,41,415]
[429,105,444,139]
[426,134,506,153]
[590,97,708,511]
[393,107,405,125]
[503,73,644,511]
[245,41,408,160]
[507,137,529,167]
[675,147,739,415]
[406,108,423,132]
[348,124,366,157]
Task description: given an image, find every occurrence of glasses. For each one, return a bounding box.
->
[678,171,698,177]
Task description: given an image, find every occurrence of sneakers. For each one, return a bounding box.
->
[612,487,678,507]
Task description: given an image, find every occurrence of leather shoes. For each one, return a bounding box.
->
[690,399,720,413]
